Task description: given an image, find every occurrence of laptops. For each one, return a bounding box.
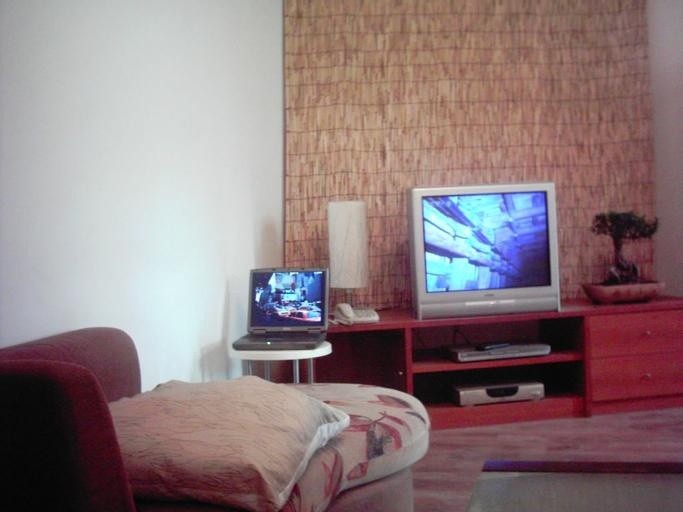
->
[233,266,329,350]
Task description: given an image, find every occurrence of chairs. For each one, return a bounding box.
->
[0,326,431,512]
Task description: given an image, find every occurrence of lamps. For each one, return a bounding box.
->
[327,199,373,307]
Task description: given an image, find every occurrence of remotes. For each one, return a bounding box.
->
[477,341,510,350]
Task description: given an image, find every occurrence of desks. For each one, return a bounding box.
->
[228,339,333,383]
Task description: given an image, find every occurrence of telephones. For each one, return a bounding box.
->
[335,303,379,325]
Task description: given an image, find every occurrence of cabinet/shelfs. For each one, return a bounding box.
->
[320,293,683,430]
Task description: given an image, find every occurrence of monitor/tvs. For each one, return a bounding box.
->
[407,182,560,320]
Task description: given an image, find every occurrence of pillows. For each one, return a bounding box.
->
[107,376,353,512]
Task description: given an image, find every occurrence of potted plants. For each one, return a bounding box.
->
[579,210,665,305]
